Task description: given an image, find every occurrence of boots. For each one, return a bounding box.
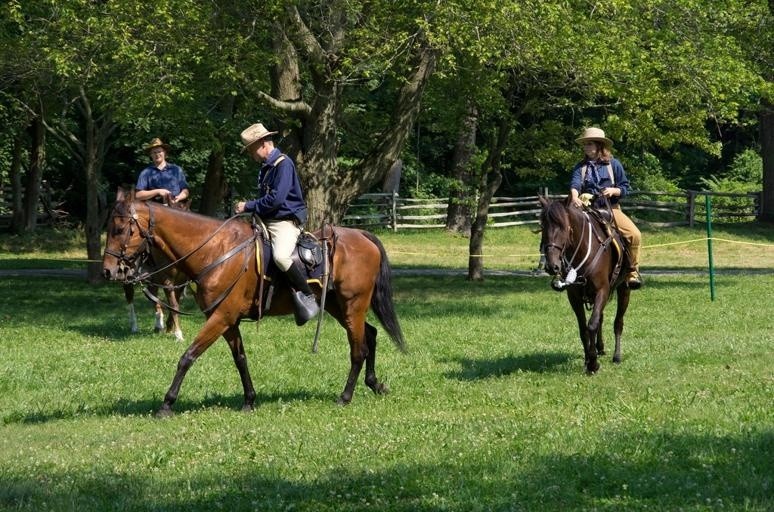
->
[284,262,317,301]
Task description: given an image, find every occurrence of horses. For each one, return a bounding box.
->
[534,190,631,377]
[115,181,193,345]
[101,184,413,420]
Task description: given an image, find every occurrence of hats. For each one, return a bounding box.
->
[239,123,278,153]
[575,128,613,147]
[144,138,171,151]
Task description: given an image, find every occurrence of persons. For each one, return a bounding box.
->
[134,137,189,207]
[544,127,643,289]
[233,122,320,321]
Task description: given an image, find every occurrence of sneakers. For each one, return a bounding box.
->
[628,277,640,287]
